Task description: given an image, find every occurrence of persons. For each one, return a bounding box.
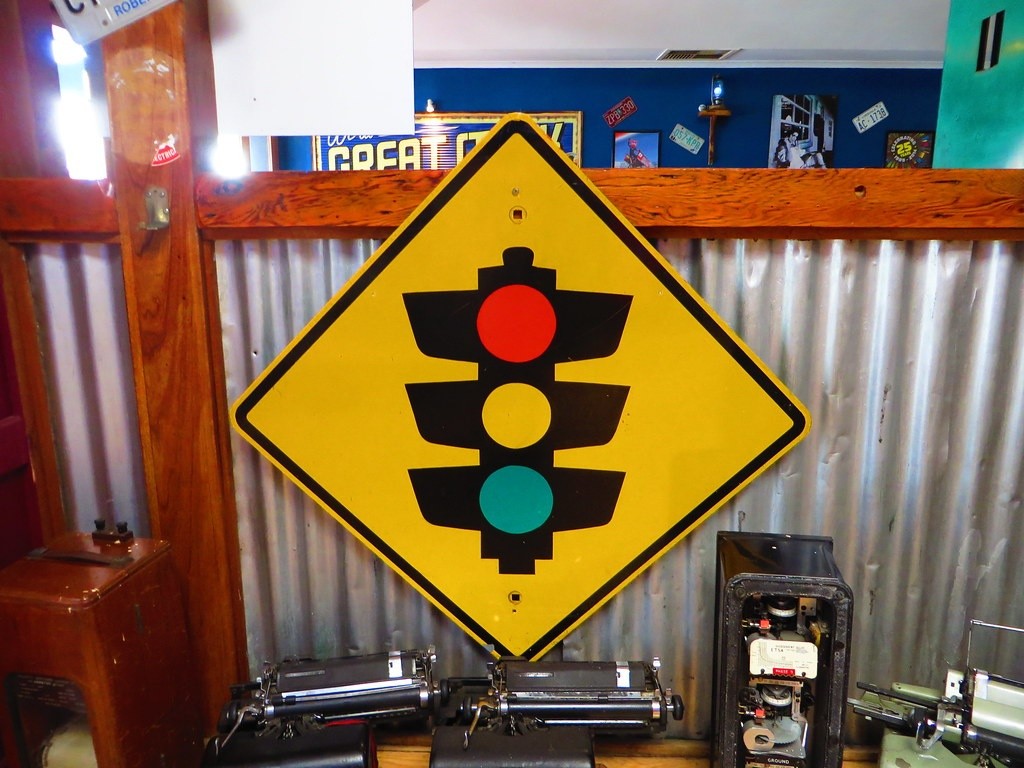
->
[778,131,826,169]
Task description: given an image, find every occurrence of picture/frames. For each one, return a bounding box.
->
[612,129,661,168]
[312,110,582,170]
[884,130,935,169]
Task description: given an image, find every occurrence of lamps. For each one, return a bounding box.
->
[708,74,725,109]
[427,99,437,114]
